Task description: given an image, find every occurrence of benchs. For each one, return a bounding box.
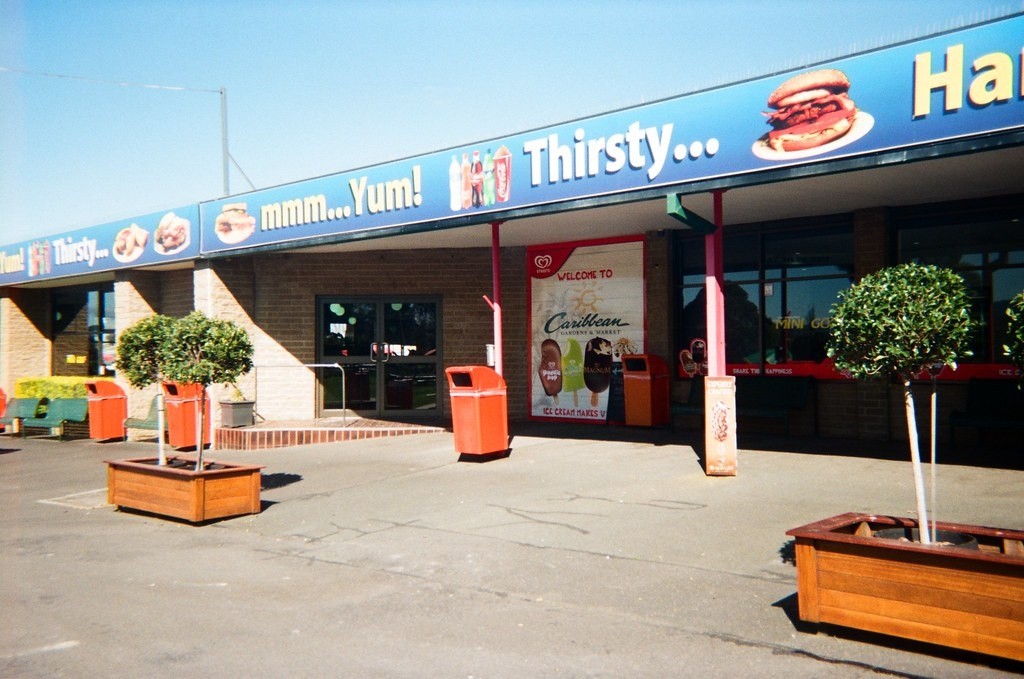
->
[0,397,50,438]
[123,393,168,443]
[23,397,89,442]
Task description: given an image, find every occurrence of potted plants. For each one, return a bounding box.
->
[103,310,268,523]
[219,382,256,427]
[784,263,1024,666]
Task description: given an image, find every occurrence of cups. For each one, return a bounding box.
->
[493,153,512,202]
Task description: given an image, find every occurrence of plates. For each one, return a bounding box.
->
[153,220,191,255]
[752,111,875,160]
[215,228,252,244]
[113,241,144,263]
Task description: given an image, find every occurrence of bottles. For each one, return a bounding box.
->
[28,241,43,276]
[482,148,495,207]
[44,240,50,273]
[449,156,461,211]
[471,150,484,208]
[461,154,471,209]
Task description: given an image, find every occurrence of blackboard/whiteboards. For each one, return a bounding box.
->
[605,361,625,421]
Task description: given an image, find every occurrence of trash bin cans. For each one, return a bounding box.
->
[0,388,7,433]
[85,381,127,440]
[161,381,211,448]
[621,353,671,427]
[445,366,509,455]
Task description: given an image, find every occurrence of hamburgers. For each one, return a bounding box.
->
[763,68,858,150]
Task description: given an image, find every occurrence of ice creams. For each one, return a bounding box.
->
[537,335,613,407]
